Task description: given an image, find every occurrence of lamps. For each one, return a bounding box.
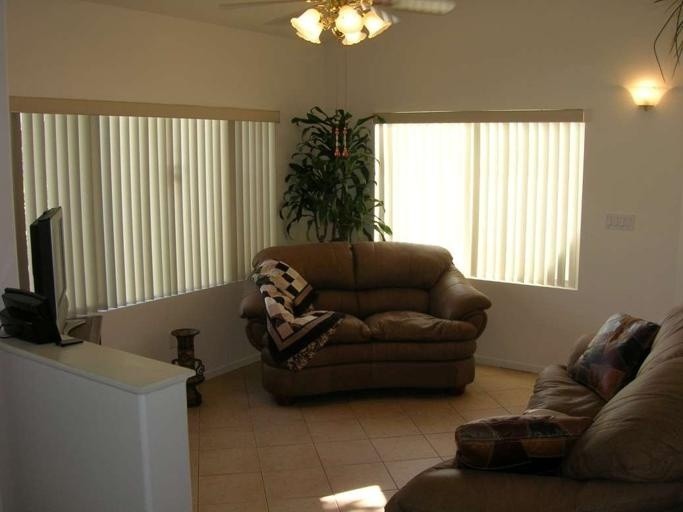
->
[291,0,391,159]
[626,81,667,111]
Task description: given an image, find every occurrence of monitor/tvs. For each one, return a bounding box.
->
[30,207,69,337]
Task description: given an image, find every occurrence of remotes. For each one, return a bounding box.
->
[60,338,83,346]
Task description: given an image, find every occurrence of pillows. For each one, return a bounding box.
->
[446,411,591,479]
[570,309,661,402]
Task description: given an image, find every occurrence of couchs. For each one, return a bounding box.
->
[238,240,494,408]
[372,303,683,511]
